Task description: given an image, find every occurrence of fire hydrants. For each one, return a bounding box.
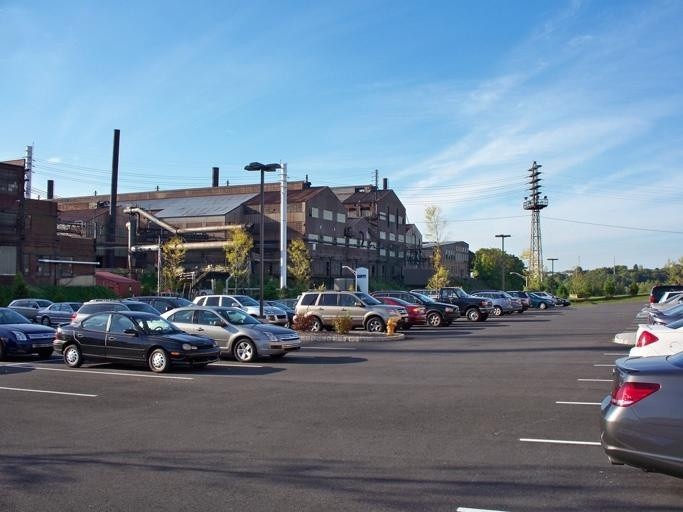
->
[384,318,397,337]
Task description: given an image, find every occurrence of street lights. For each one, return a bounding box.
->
[494,234,511,290]
[244,161,281,320]
[546,258,558,295]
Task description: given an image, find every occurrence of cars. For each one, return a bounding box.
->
[70,296,194,330]
[51,311,221,375]
[598,283,681,480]
[191,295,288,327]
[6,299,54,319]
[35,301,80,327]
[0,307,57,361]
[159,305,300,364]
